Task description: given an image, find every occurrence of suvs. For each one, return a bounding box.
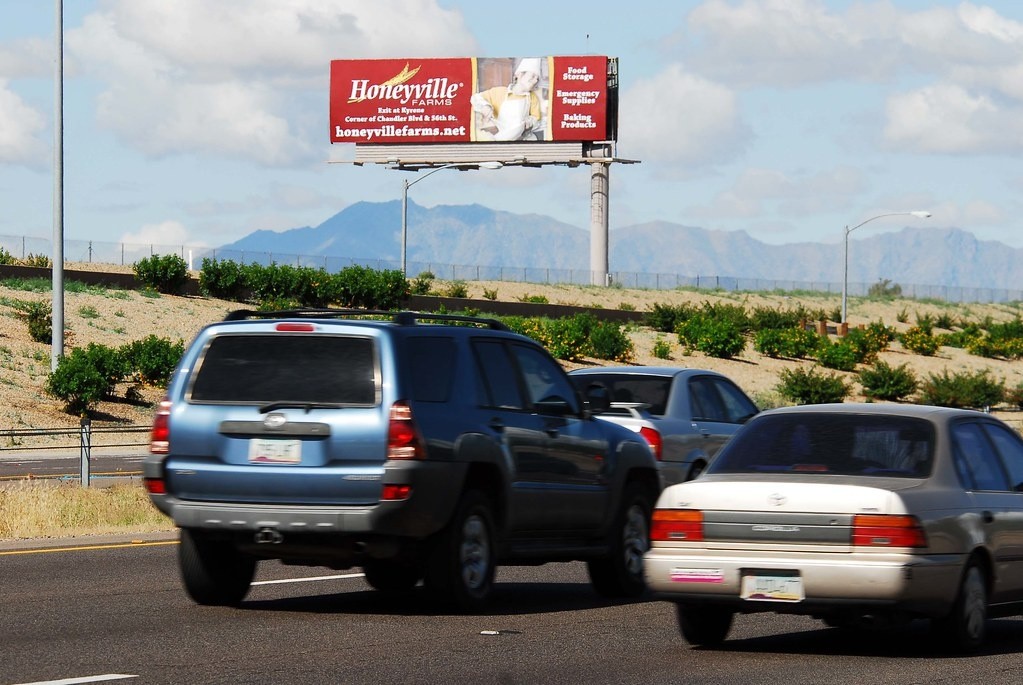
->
[142,311,663,609]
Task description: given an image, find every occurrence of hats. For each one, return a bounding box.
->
[514,57,541,84]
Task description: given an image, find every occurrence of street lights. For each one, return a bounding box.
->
[400,161,503,284]
[840,210,932,324]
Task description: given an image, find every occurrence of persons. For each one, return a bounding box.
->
[468,56,543,142]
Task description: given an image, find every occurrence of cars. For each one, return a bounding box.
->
[642,403,1022,653]
[533,368,759,487]
